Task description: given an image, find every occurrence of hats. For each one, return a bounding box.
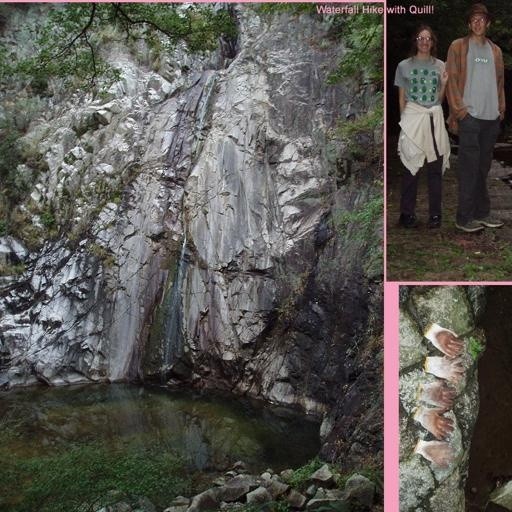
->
[463,5,495,23]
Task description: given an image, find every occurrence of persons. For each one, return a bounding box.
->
[445,2,508,233]
[392,24,447,230]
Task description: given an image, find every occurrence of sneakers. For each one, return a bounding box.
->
[429,215,441,228]
[400,214,417,227]
[455,222,485,232]
[473,216,505,228]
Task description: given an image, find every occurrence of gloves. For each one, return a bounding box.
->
[424,356,466,385]
[423,324,463,357]
[414,440,456,469]
[413,406,455,440]
[415,381,455,408]
